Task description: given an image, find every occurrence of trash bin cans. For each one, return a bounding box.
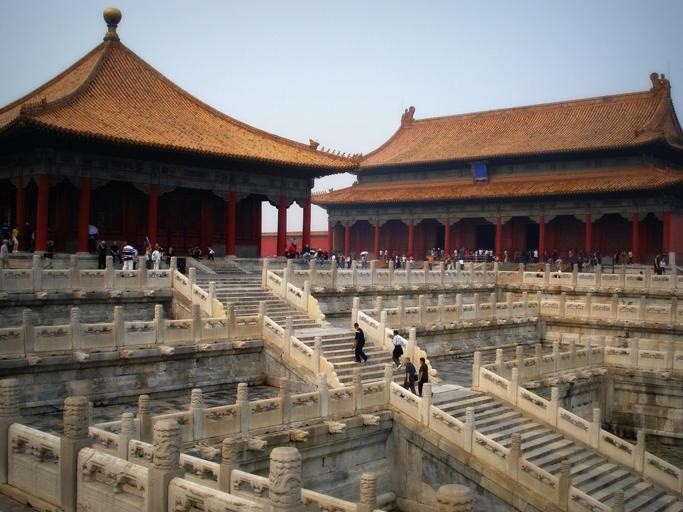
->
[176,258,186,273]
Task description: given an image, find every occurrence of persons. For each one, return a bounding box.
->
[351,322,367,363]
[10,225,18,252]
[88,224,214,277]
[0,239,9,269]
[284,235,667,279]
[389,330,403,368]
[418,357,433,398]
[400,357,416,399]
